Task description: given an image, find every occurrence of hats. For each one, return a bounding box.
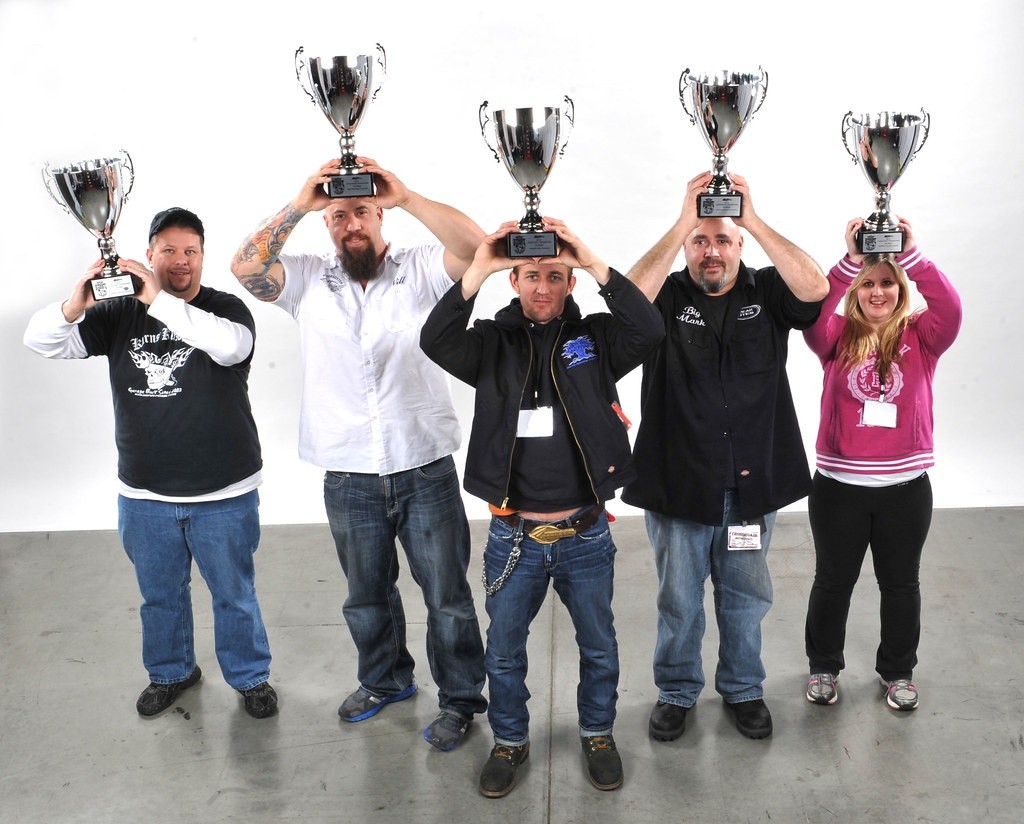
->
[149,207,205,242]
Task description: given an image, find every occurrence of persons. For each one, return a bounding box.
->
[229,154,489,752]
[418,215,667,798]
[623,171,831,742]
[799,214,963,711]
[22,207,278,719]
[41,148,143,301]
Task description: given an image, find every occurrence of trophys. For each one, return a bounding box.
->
[478,95,575,258]
[842,107,930,255]
[679,64,769,218]
[294,42,387,198]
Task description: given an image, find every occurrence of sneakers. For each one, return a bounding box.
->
[880,676,919,710]
[239,681,277,719]
[724,698,773,738]
[337,679,418,723]
[649,699,686,741]
[807,673,837,705]
[136,664,202,717]
[479,742,530,797]
[581,735,624,790]
[424,710,473,751]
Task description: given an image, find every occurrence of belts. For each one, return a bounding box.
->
[496,504,603,544]
